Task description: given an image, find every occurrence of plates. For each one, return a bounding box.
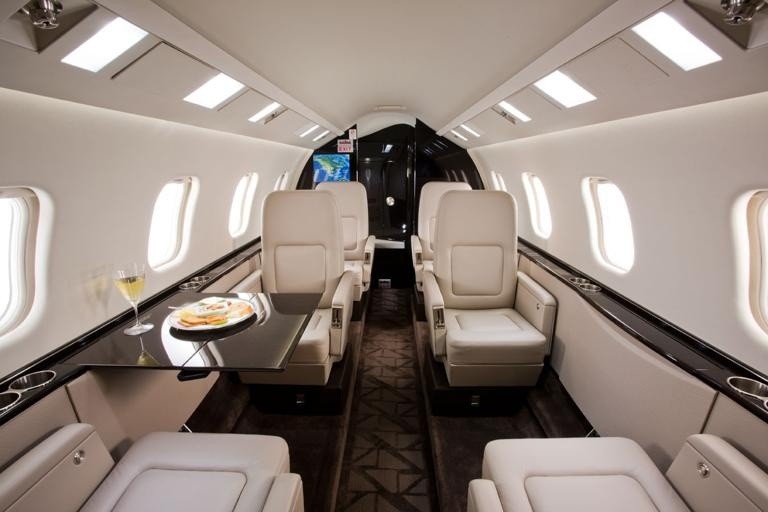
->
[168,313,259,343]
[167,295,255,333]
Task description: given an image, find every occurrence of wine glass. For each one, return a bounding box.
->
[111,267,154,337]
[135,335,162,367]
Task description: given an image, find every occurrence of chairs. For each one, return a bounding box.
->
[225,180,377,389]
[2,412,305,512]
[464,433,768,512]
[409,180,558,391]
